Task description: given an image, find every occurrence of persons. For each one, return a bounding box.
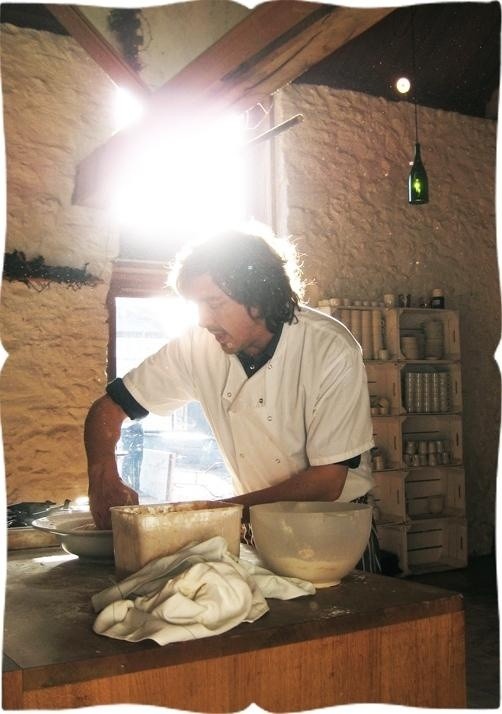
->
[84,232,382,574]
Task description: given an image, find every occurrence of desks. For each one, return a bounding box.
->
[3,542,468,708]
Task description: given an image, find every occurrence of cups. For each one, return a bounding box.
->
[302,279,451,469]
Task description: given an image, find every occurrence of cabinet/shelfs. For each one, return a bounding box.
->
[320,303,468,577]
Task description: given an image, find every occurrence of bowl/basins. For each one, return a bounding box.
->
[32,511,118,565]
[250,501,373,588]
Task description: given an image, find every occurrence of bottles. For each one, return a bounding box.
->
[407,142,431,206]
